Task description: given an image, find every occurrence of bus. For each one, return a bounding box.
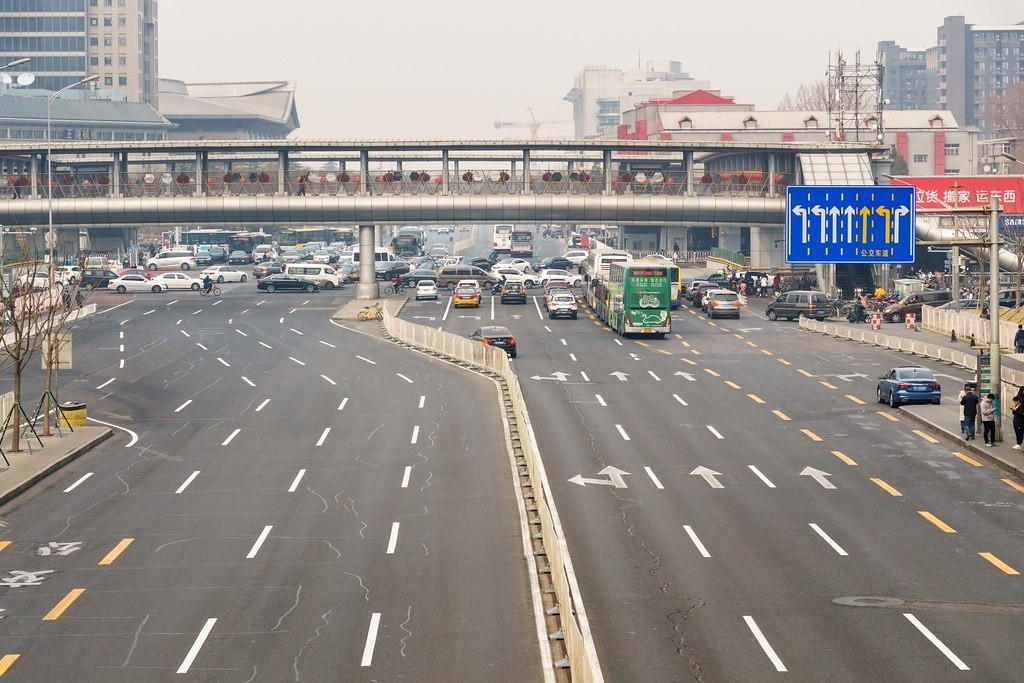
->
[581,249,769,336]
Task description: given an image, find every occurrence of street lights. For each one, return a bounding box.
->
[882,171,960,314]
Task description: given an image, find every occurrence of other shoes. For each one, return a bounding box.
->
[984,443,991,447]
[765,293,768,297]
[759,293,762,298]
[993,442,999,446]
[1012,444,1022,449]
[965,434,971,441]
[970,437,976,439]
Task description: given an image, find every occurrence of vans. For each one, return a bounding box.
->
[17,256,123,290]
[882,291,954,322]
[765,290,831,322]
[282,263,345,290]
[145,251,197,271]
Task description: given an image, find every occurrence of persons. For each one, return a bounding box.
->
[723,264,811,297]
[298,174,312,196]
[392,275,401,294]
[63,290,84,312]
[908,266,990,315]
[1014,325,1024,353]
[138,243,160,266]
[203,275,213,294]
[958,384,978,441]
[856,286,887,313]
[1010,386,1024,449]
[12,179,20,199]
[980,393,999,447]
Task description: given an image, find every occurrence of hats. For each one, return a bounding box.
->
[965,386,971,390]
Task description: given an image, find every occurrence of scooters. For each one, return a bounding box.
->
[829,290,910,324]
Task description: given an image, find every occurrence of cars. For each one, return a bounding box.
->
[162,229,272,265]
[107,268,204,294]
[257,274,321,293]
[336,224,500,291]
[199,266,247,284]
[384,280,482,308]
[491,224,590,319]
[48,74,100,428]
[468,326,517,358]
[876,365,941,408]
[253,227,353,278]
[938,289,1024,310]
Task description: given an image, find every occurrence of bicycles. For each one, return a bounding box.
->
[357,302,384,322]
[199,282,221,296]
